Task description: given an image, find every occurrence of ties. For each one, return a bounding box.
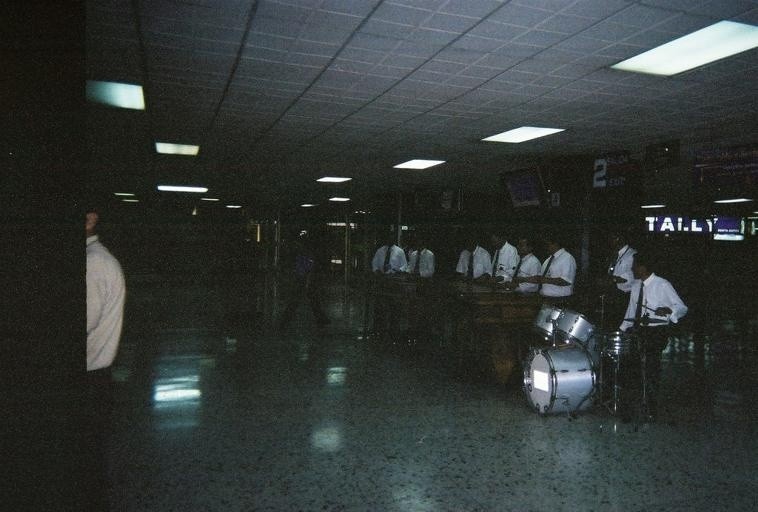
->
[414,252,420,272]
[467,254,473,277]
[492,251,499,277]
[513,260,521,277]
[384,248,390,272]
[538,255,554,289]
[633,282,644,329]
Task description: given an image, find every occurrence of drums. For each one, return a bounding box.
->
[586,333,608,367]
[554,304,598,349]
[531,300,561,341]
[606,331,636,355]
[522,341,598,416]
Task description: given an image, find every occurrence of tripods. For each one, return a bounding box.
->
[572,293,654,424]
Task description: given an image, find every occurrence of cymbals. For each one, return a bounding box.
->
[580,273,627,284]
[623,317,666,324]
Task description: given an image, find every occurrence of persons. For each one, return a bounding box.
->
[364,227,703,419]
[84,194,127,512]
[280,227,338,324]
